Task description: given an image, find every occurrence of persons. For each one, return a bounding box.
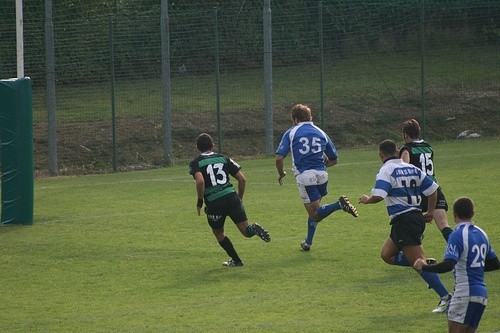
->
[357,139,453,312]
[275,104,359,250]
[413,197,500,333]
[396,119,453,243]
[189,133,271,267]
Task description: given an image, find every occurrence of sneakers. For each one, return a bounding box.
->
[222,259,244,267]
[301,240,311,250]
[426,258,437,265]
[338,195,359,218]
[432,293,452,313]
[252,222,272,243]
